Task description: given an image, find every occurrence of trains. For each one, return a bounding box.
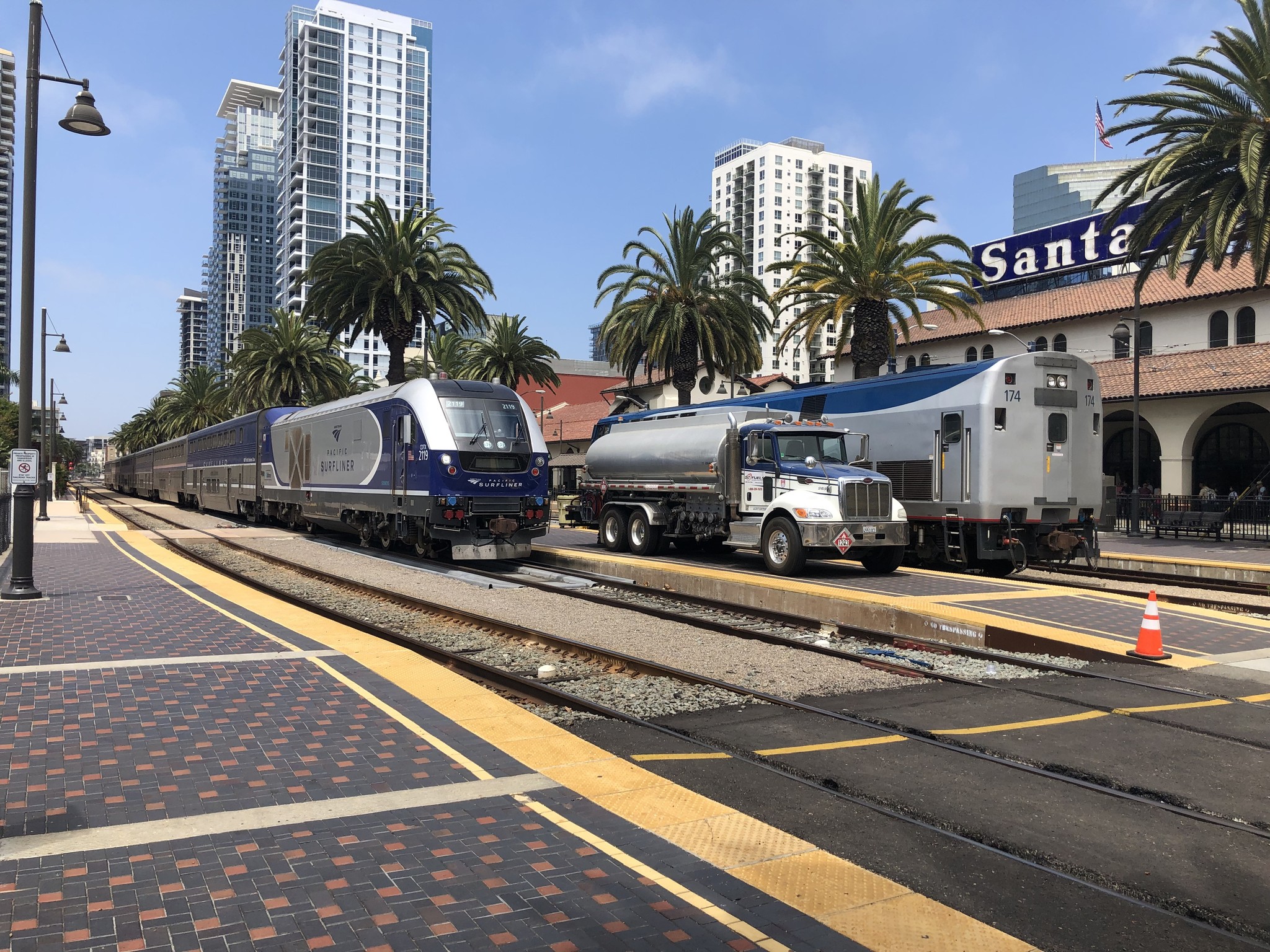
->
[103,372,553,561]
[588,350,1104,580]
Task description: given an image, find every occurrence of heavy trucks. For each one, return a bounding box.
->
[564,404,909,578]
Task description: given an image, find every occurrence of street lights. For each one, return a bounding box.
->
[885,323,938,375]
[35,306,73,522]
[532,395,554,435]
[988,329,1036,353]
[552,419,562,495]
[615,396,650,409]
[1110,294,1144,537]
[47,378,69,502]
[0,0,111,601]
[405,337,445,379]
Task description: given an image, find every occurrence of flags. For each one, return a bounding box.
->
[1095,97,1113,149]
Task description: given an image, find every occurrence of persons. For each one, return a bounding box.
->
[1116,479,1162,520]
[1191,480,1270,522]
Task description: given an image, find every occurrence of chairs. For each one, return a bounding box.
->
[785,440,804,458]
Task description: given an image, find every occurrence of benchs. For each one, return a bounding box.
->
[1146,510,1227,543]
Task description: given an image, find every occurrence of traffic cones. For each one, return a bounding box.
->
[1126,591,1172,660]
